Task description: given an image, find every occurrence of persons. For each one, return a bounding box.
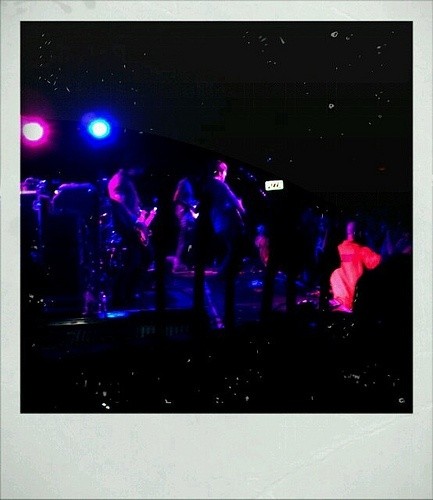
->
[20,144,414,413]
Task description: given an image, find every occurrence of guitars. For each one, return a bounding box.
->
[132,206,159,247]
